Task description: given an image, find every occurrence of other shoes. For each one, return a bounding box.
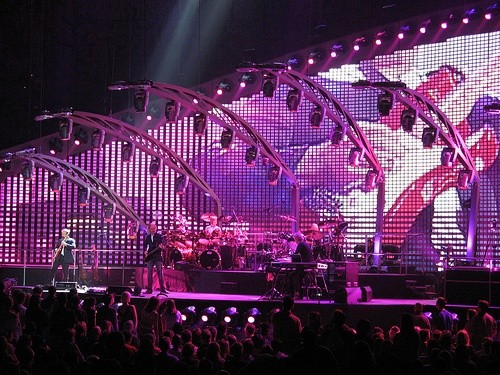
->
[161,288,170,293]
[143,289,152,294]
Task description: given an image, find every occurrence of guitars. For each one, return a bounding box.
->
[51,232,68,270]
[143,240,167,262]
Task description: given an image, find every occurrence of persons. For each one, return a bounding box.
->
[144,223,169,294]
[47,229,76,282]
[206,216,221,234]
[293,233,312,263]
[0,286,500,375]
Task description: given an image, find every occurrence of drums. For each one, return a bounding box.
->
[169,248,186,261]
[256,242,273,258]
[200,249,221,270]
[198,237,208,246]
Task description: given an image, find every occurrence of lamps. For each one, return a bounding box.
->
[104,203,116,222]
[175,175,189,194]
[49,139,63,154]
[194,112,207,135]
[240,5,497,189]
[75,128,88,145]
[146,105,159,121]
[134,91,149,113]
[216,80,230,95]
[92,129,105,151]
[150,157,161,178]
[193,89,207,104]
[78,188,90,206]
[181,306,259,324]
[21,161,34,178]
[129,221,139,239]
[0,160,11,173]
[58,119,72,140]
[221,131,235,149]
[165,102,180,123]
[122,142,135,162]
[50,173,63,192]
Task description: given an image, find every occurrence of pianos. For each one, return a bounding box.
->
[270,262,328,298]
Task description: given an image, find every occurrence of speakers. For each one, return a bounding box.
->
[335,287,363,304]
[55,281,78,289]
[106,285,135,295]
[360,286,373,303]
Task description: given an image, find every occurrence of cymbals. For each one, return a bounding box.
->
[303,228,324,241]
[318,220,337,228]
[200,213,218,223]
[279,214,297,223]
[333,223,349,236]
[234,217,243,222]
[220,215,232,222]
